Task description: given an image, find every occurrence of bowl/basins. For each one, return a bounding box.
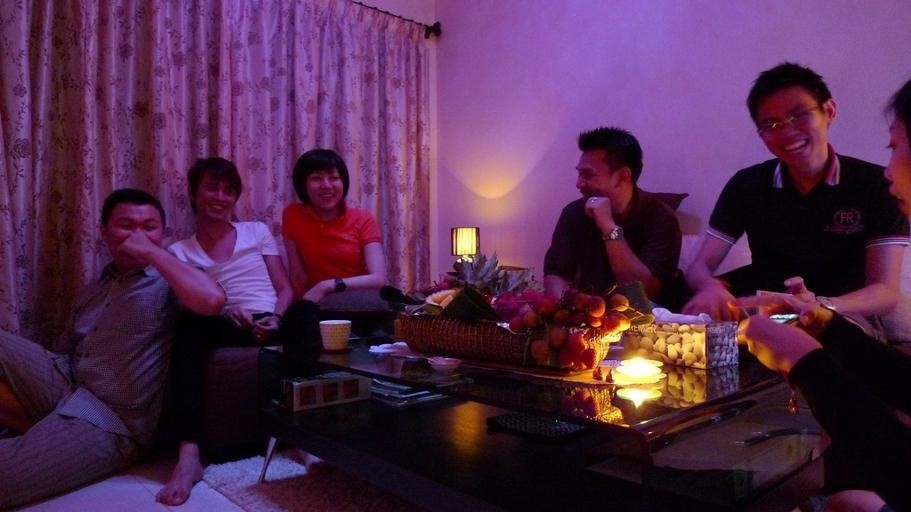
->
[426,357,463,375]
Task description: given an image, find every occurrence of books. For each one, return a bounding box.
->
[368,370,477,408]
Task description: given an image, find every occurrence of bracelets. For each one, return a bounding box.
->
[271,312,283,321]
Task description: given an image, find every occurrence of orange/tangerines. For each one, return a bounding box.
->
[510,295,632,369]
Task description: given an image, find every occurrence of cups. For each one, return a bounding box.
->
[320,319,352,351]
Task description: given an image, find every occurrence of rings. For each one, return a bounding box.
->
[591,200,594,204]
[256,333,262,338]
[588,197,597,200]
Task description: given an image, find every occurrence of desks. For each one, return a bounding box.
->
[252,345,826,511]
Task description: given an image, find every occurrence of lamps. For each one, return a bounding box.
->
[450,225,481,272]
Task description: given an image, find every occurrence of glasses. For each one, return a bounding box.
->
[755,107,823,141]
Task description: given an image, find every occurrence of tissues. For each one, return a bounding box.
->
[624,307,739,370]
[644,371,740,409]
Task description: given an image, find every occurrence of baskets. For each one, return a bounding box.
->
[393,316,613,366]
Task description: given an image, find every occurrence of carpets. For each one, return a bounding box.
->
[200,456,409,512]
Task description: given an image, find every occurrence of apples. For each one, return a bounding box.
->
[484,289,545,321]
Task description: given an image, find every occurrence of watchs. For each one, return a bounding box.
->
[815,295,837,312]
[334,277,347,293]
[601,226,625,241]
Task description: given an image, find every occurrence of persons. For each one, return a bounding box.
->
[0,187,225,512]
[280,145,393,348]
[736,79,911,512]
[678,63,911,343]
[154,156,323,505]
[542,125,683,320]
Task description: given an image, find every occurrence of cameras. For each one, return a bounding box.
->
[766,311,801,328]
